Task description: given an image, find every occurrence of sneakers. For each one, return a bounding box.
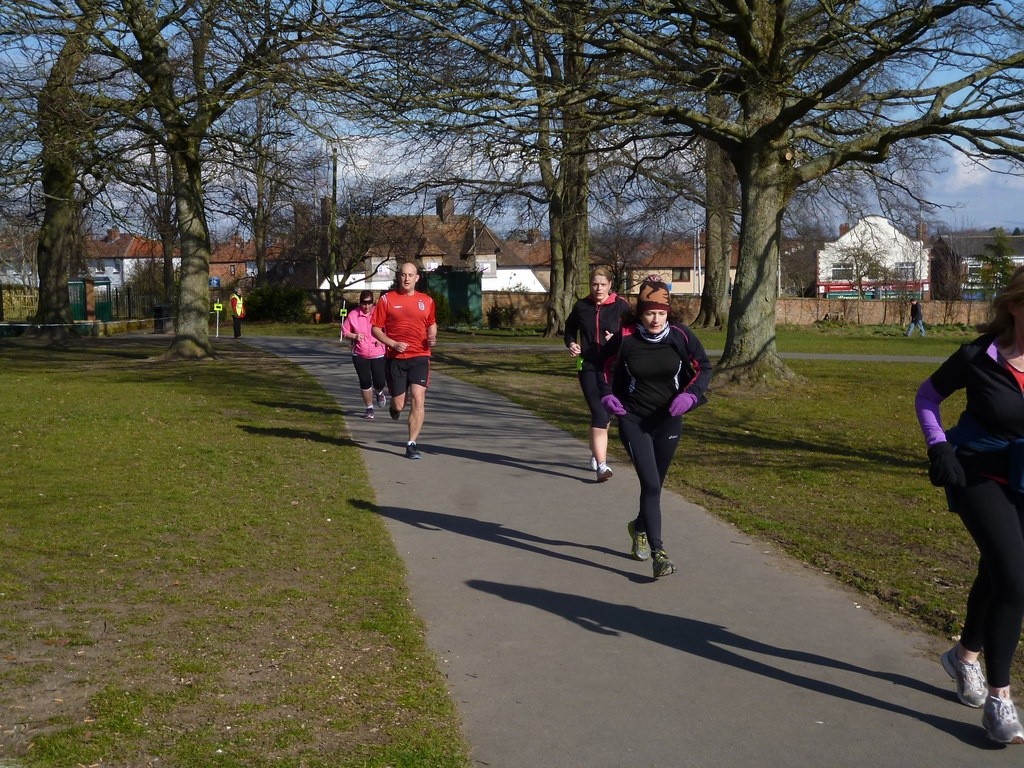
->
[375,391,386,408]
[941,644,988,708]
[982,695,1024,744]
[597,465,613,483]
[389,407,400,420]
[628,521,649,560]
[406,442,421,458]
[363,408,374,418]
[651,548,676,578]
[591,456,597,471]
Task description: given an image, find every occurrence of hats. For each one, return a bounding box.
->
[637,281,672,313]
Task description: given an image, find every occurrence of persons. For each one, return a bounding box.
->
[341,290,386,419]
[902,299,926,338]
[564,268,635,482]
[230,288,245,339]
[916,265,1024,744]
[370,262,437,459]
[598,273,714,579]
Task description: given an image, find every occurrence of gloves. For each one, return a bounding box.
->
[927,442,966,488]
[670,393,697,416]
[601,394,626,415]
